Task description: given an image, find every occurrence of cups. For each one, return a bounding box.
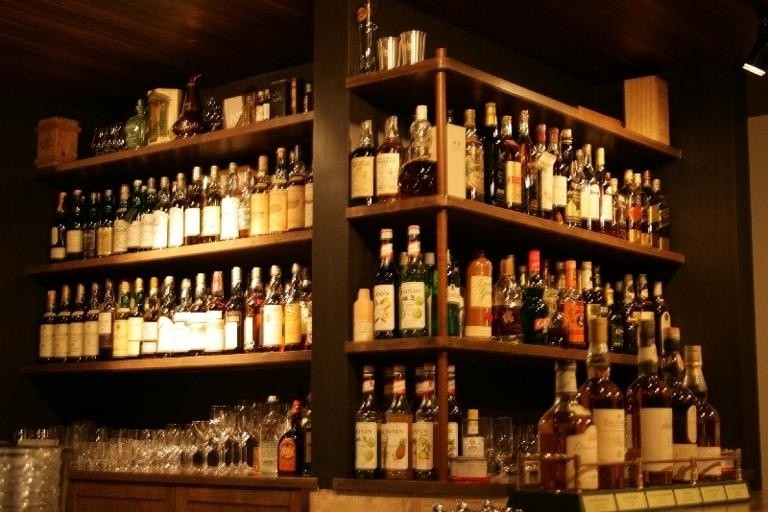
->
[15,419,166,475]
[376,36,403,72]
[399,30,427,67]
[723,448,742,483]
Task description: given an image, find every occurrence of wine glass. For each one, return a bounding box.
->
[166,399,260,475]
[89,120,125,154]
[481,414,537,475]
[201,93,223,133]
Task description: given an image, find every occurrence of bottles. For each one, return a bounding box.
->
[636,273,654,343]
[351,224,438,341]
[448,363,463,456]
[536,359,599,491]
[35,261,311,362]
[349,119,375,207]
[49,170,185,261]
[445,246,583,348]
[398,106,437,197]
[651,276,671,355]
[185,145,313,245]
[660,327,699,485]
[376,114,407,204]
[260,392,311,476]
[447,97,671,250]
[594,266,605,304]
[623,274,638,355]
[604,288,614,312]
[608,281,624,352]
[584,259,600,347]
[243,89,270,127]
[353,361,437,480]
[463,408,486,459]
[681,345,722,485]
[358,2,378,73]
[300,82,313,113]
[577,317,625,488]
[626,319,673,486]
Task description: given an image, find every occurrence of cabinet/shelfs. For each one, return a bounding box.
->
[22,112,312,381]
[67,473,319,511]
[332,51,690,495]
